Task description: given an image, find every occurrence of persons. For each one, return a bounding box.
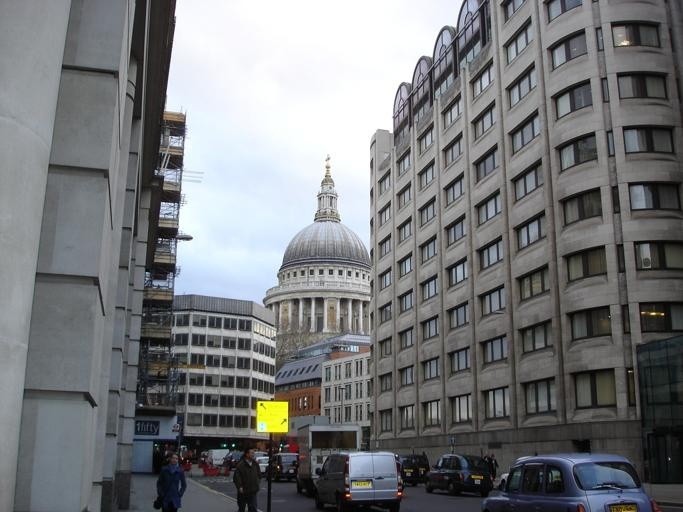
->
[230,447,261,512]
[482,451,500,481]
[154,452,187,512]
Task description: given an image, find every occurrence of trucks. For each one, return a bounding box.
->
[296,422,364,496]
[312,451,403,511]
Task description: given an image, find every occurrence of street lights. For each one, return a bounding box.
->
[168,231,195,407]
[450,435,457,454]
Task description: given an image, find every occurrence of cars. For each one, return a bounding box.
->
[199,447,298,482]
[393,452,663,512]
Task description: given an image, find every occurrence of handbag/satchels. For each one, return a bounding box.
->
[154,497,162,509]
